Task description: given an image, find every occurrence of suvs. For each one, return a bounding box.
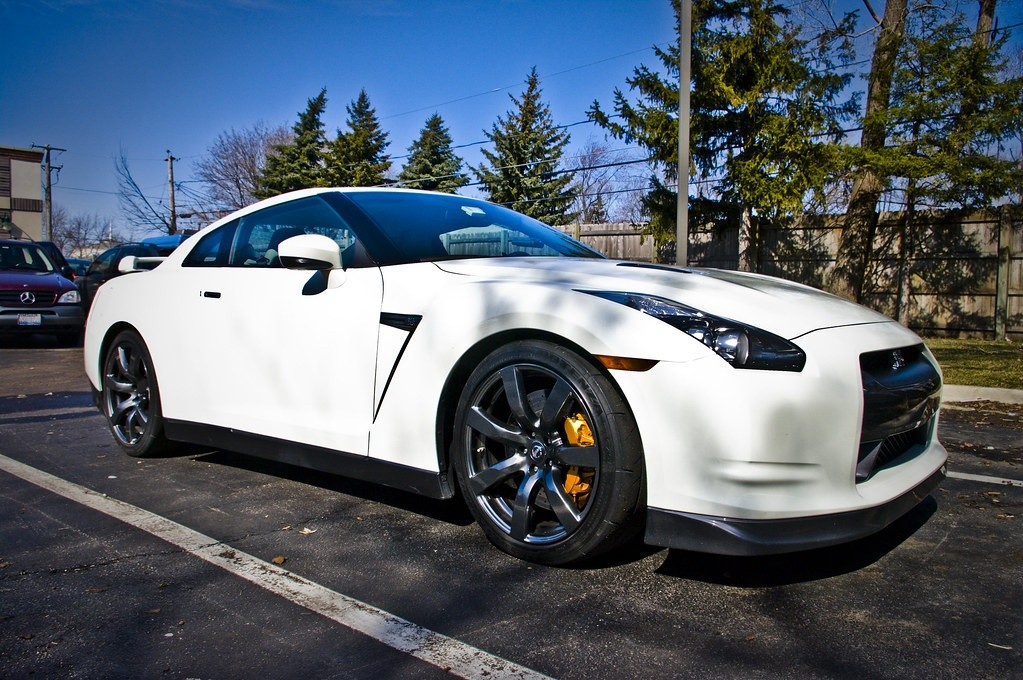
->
[72,241,169,331]
[0,238,82,346]
[30,240,75,284]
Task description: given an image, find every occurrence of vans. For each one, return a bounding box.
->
[140,234,191,252]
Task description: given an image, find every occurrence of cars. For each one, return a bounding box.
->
[65,258,94,278]
[81,184,952,568]
